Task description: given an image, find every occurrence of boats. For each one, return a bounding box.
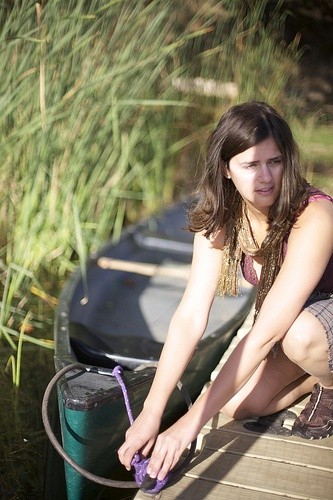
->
[41,196,261,499]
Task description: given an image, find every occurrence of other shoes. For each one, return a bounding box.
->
[291,382,333,440]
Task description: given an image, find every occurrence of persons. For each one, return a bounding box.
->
[116,101,333,481]
